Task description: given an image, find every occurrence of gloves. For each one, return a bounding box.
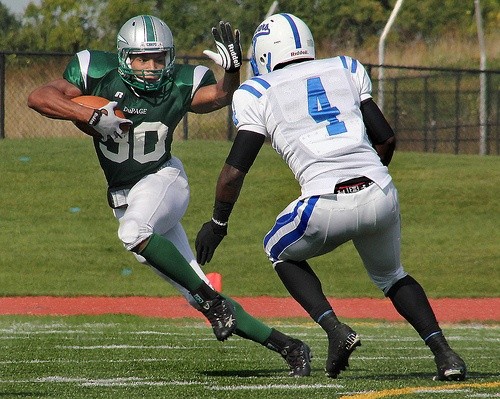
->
[88,102,134,142]
[202,20,243,74]
[194,208,230,266]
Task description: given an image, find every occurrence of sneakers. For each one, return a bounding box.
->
[326,325,361,379]
[283,339,312,378]
[428,359,468,381]
[203,294,238,341]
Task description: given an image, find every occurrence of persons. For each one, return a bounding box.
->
[196,11,466,380]
[27,16,313,376]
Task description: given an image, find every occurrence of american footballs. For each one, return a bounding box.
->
[67,95,126,137]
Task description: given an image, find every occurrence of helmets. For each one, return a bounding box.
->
[248,13,315,75]
[117,15,176,97]
